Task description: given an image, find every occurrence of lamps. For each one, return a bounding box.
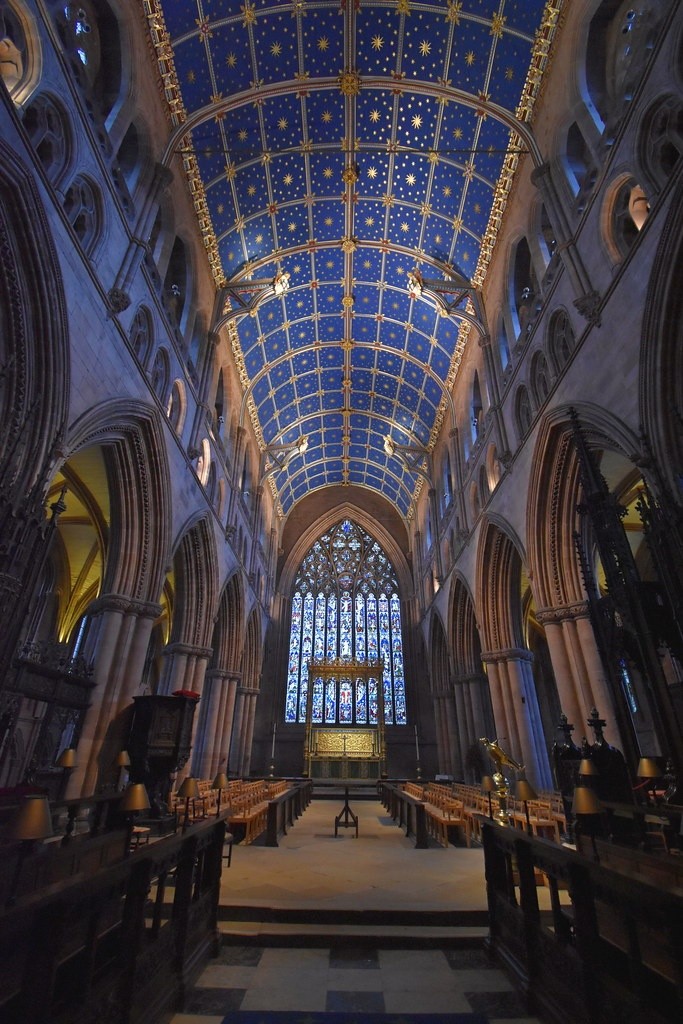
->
[578,759,600,786]
[55,748,80,800]
[211,773,229,816]
[482,776,500,821]
[0,795,54,911]
[176,777,196,833]
[571,787,607,864]
[636,757,664,801]
[117,751,131,792]
[117,784,151,861]
[515,781,538,836]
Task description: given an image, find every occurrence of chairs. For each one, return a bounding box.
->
[400,782,567,848]
[167,780,295,845]
[128,824,151,853]
